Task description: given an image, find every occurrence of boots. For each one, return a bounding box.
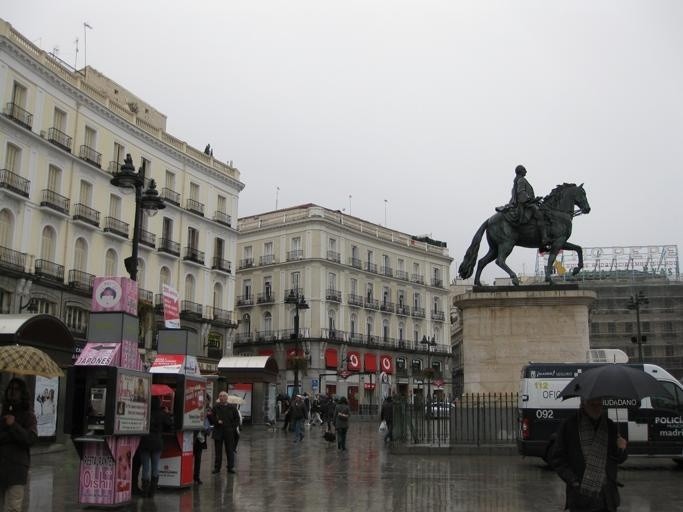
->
[140,478,149,497]
[150,474,158,497]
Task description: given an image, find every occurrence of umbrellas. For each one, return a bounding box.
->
[0,343,65,419]
[151,384,175,396]
[554,353,674,462]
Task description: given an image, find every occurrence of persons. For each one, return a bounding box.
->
[545,397,628,512]
[512,165,555,246]
[0,378,38,512]
[193,390,243,484]
[278,391,351,451]
[131,397,173,498]
[381,396,393,443]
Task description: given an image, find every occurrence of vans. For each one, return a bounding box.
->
[516,348,682,475]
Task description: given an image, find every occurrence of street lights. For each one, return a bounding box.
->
[282,288,310,399]
[109,152,167,282]
[419,333,438,418]
[626,291,649,363]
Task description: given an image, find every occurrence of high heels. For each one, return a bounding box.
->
[192,476,202,484]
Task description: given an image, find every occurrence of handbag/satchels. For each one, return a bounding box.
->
[338,407,348,421]
[324,423,336,442]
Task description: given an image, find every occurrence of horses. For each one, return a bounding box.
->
[457,183,591,286]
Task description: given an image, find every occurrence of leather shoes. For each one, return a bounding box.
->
[299,433,304,441]
[227,469,235,474]
[133,488,142,495]
[212,469,220,473]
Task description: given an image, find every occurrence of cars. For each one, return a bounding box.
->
[423,401,454,422]
